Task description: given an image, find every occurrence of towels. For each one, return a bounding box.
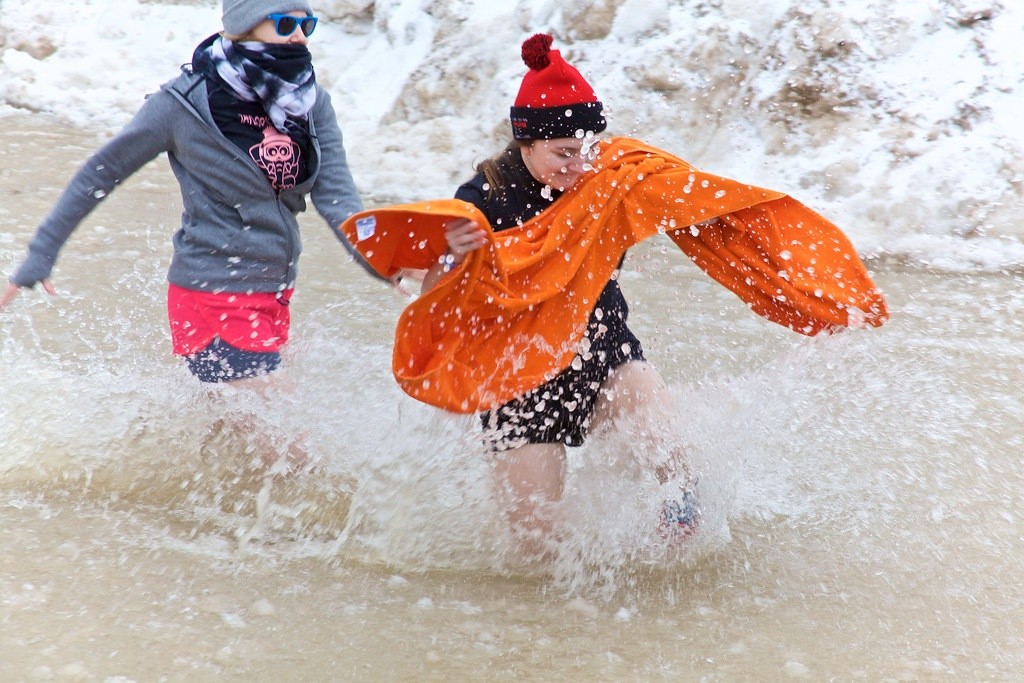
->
[335,135,890,416]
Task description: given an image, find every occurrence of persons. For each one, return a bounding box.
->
[0,1,431,413]
[416,31,699,578]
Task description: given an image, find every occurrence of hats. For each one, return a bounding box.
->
[510,32,605,141]
[222,0,313,37]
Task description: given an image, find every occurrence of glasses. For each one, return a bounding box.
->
[266,14,320,37]
